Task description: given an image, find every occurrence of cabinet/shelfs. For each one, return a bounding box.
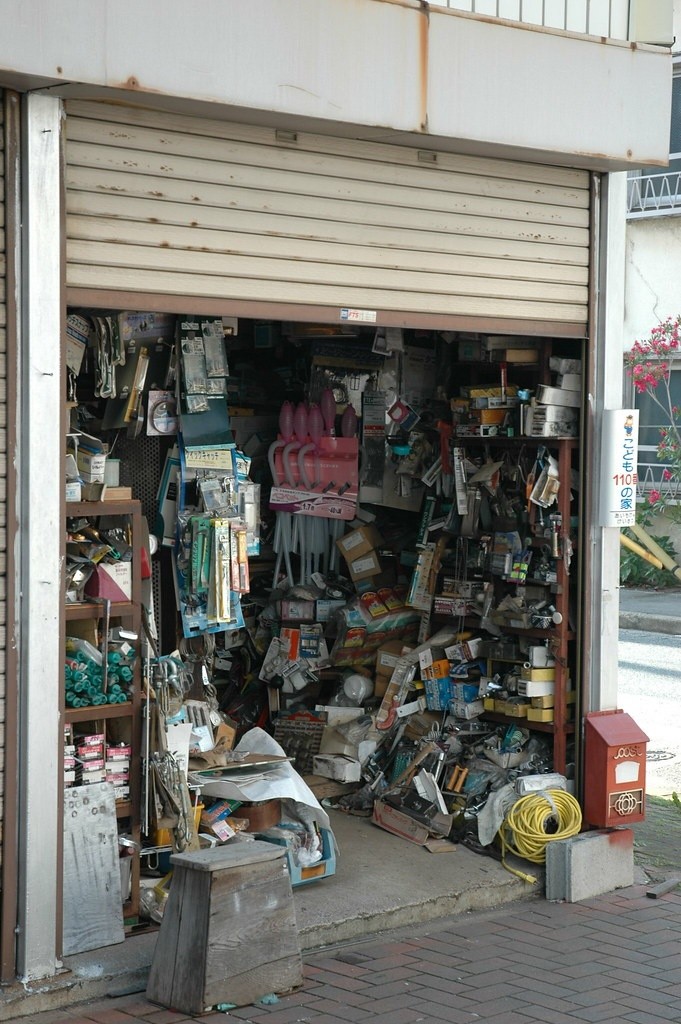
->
[61,500,143,918]
[431,437,579,775]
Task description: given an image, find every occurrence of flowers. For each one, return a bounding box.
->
[625,314,681,507]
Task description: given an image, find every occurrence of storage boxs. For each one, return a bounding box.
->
[347,545,396,595]
[374,640,417,700]
[336,525,384,562]
